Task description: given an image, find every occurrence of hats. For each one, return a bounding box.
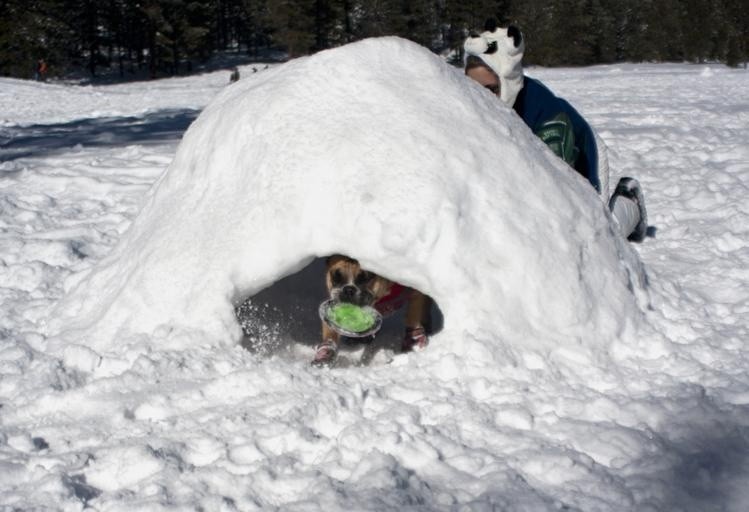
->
[463,18,526,109]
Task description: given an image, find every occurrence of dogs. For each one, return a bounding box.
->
[310,255,429,372]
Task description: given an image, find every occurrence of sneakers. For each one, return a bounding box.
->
[612,177,647,244]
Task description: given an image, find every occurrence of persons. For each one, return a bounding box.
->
[30,56,47,82]
[460,17,649,243]
[230,67,239,82]
